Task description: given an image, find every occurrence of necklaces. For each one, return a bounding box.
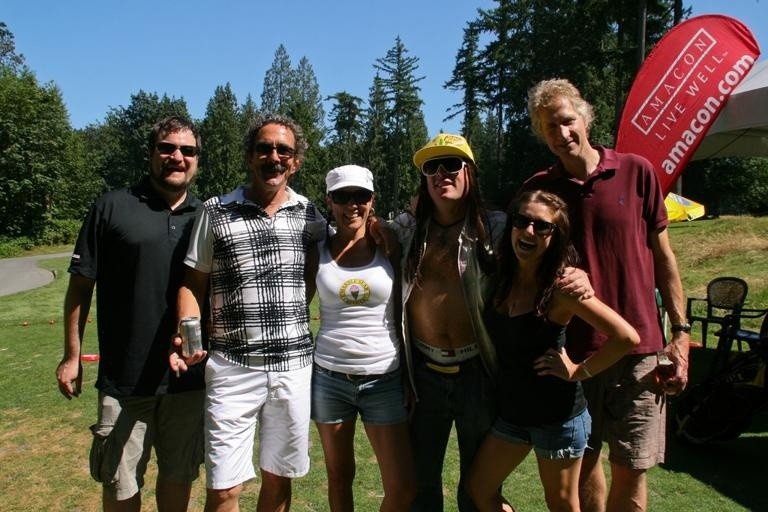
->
[433,217,464,248]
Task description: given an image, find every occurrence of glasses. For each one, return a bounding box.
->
[328,189,373,204]
[514,211,556,236]
[422,156,467,178]
[256,141,294,157]
[156,142,200,157]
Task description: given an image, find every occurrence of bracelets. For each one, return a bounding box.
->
[580,360,593,379]
[670,324,692,332]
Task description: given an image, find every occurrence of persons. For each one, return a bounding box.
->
[384,132,595,511]
[301,164,420,511]
[167,111,394,512]
[55,119,208,512]
[522,78,692,512]
[459,190,642,512]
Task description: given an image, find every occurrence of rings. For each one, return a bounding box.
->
[548,355,553,359]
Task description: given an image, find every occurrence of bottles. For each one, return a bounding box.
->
[655,346,681,399]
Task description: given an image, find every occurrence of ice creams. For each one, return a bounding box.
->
[350,285,359,301]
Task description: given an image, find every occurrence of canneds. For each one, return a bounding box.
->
[180,316,204,358]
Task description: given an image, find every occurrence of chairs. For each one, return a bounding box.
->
[721,306,768,389]
[685,278,747,364]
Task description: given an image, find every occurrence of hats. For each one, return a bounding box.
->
[413,133,476,171]
[326,163,374,194]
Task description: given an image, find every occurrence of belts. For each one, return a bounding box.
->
[331,371,378,383]
